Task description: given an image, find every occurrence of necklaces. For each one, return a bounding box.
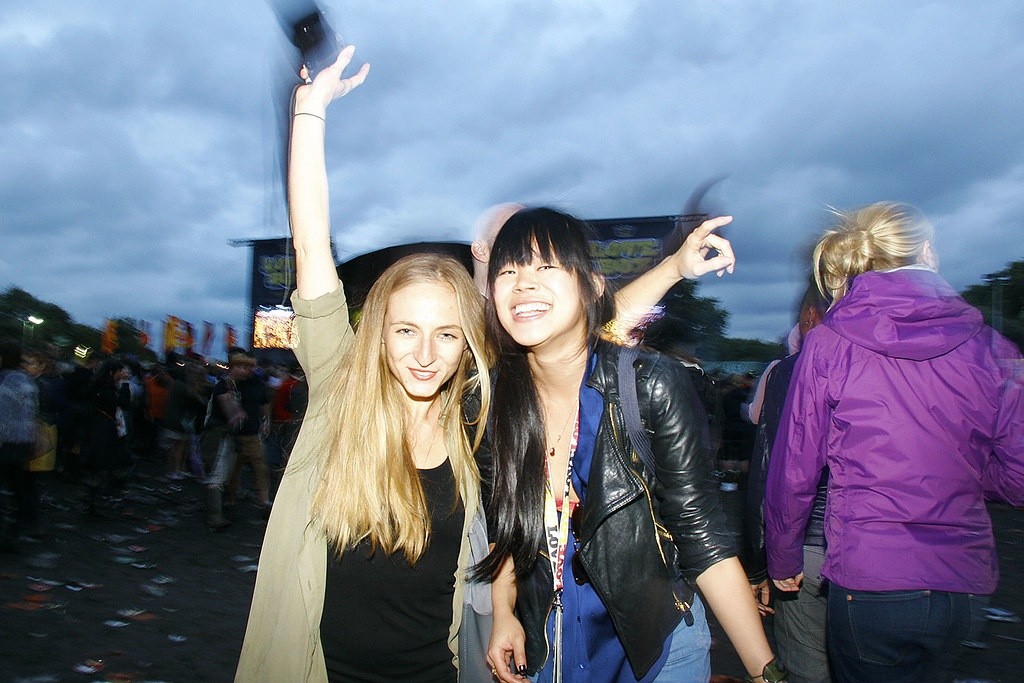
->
[527,357,590,456]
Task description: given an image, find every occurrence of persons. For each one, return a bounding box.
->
[639,272,853,682]
[762,196,1023,683]
[484,206,795,683]
[446,205,747,683]
[1,280,311,568]
[237,45,533,682]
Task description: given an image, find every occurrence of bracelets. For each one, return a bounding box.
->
[747,652,792,682]
